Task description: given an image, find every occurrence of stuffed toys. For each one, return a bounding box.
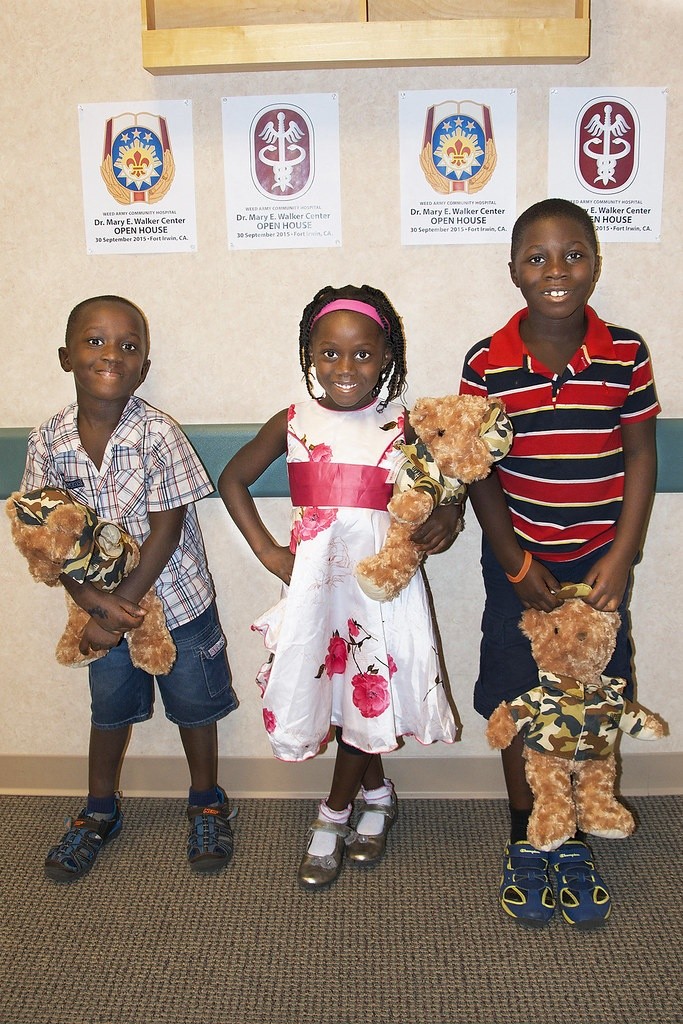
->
[4,485,177,676]
[485,582,664,852]
[356,394,513,603]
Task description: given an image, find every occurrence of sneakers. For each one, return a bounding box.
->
[43,800,123,882]
[499,837,612,930]
[186,785,240,872]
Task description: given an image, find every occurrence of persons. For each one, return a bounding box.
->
[217,286,468,889]
[459,198,662,929]
[19,295,239,880]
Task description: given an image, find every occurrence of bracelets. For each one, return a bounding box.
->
[506,549,532,583]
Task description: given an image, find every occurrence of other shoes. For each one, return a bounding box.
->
[344,791,399,863]
[296,816,350,890]
